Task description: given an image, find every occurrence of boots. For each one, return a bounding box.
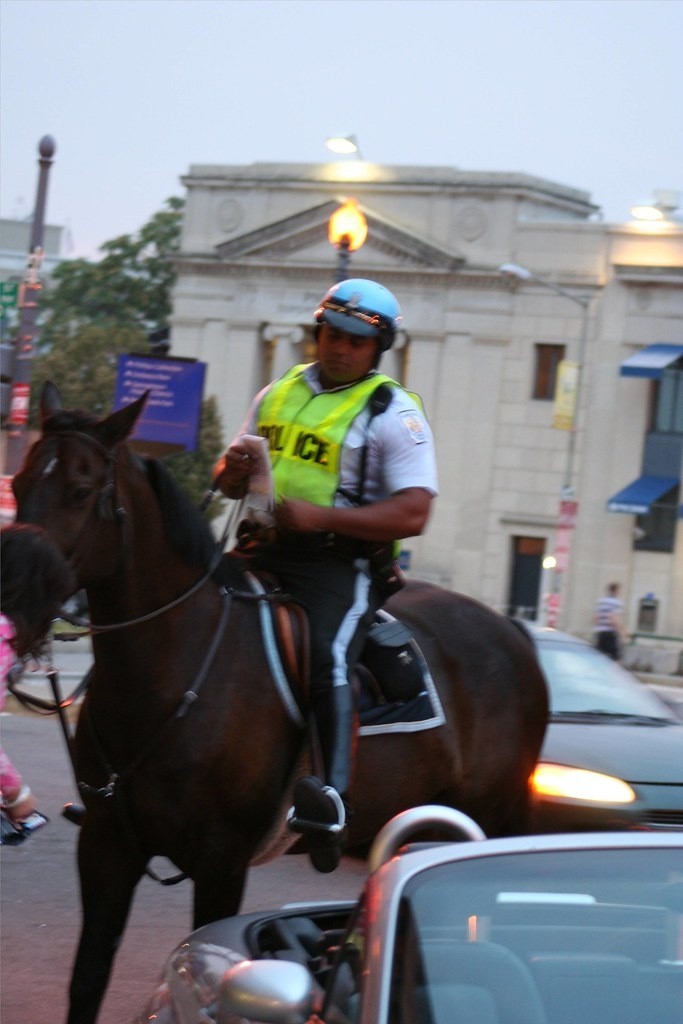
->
[289,683,356,876]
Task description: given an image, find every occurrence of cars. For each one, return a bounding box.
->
[501,619,683,829]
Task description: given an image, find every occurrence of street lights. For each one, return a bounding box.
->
[327,200,367,287]
[500,261,592,635]
[0,134,60,515]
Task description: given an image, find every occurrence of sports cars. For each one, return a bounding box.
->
[125,803,681,1024]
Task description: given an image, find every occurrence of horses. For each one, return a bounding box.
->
[0,381,552,1024]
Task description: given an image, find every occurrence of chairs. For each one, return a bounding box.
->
[324,843,548,1024]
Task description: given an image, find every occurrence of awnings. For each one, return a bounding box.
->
[607,474,682,518]
[619,343,683,379]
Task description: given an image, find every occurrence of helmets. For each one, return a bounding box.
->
[312,278,408,346]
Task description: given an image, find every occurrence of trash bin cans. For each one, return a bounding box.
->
[637,599,660,633]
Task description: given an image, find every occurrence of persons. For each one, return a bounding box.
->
[590,581,630,665]
[62,278,439,876]
[0,521,78,825]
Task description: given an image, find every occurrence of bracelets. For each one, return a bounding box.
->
[0,785,31,811]
